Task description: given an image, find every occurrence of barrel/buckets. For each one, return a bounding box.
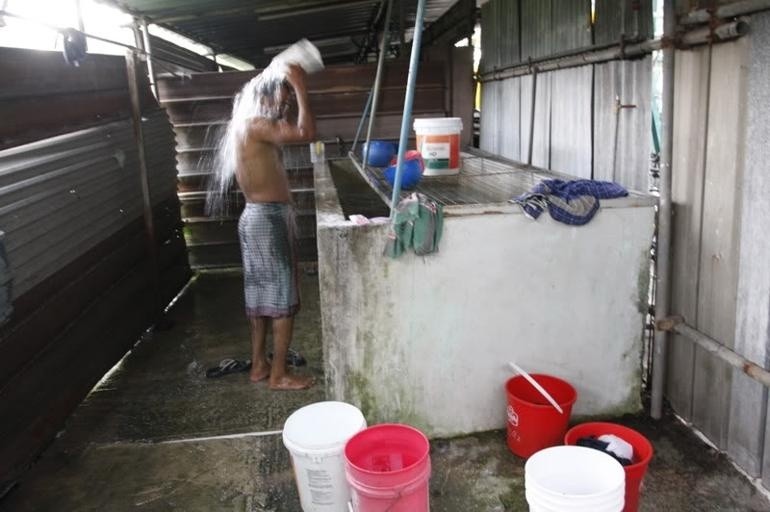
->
[282,401,367,512]
[522,447,627,512]
[501,361,578,458]
[271,38,324,78]
[412,117,465,177]
[564,422,653,510]
[342,423,433,512]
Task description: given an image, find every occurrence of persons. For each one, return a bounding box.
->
[228,62,318,389]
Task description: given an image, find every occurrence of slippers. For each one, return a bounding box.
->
[206,357,251,378]
[268,347,307,367]
